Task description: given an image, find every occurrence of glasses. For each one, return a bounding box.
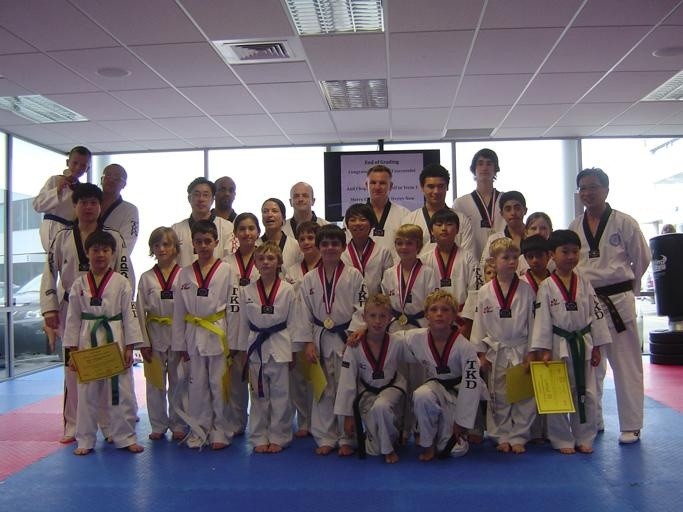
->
[101,174,126,183]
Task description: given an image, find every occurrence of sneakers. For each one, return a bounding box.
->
[618,430,640,443]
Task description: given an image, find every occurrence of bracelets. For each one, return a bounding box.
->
[127,345,134,350]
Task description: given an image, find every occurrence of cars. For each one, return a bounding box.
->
[0,275,20,301]
[0,270,58,352]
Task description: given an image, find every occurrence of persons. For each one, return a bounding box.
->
[138,148,613,462]
[31,146,92,353]
[40,183,136,443]
[63,230,144,456]
[100,162,141,423]
[569,168,653,444]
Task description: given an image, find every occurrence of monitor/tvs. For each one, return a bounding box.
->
[324,150,440,223]
